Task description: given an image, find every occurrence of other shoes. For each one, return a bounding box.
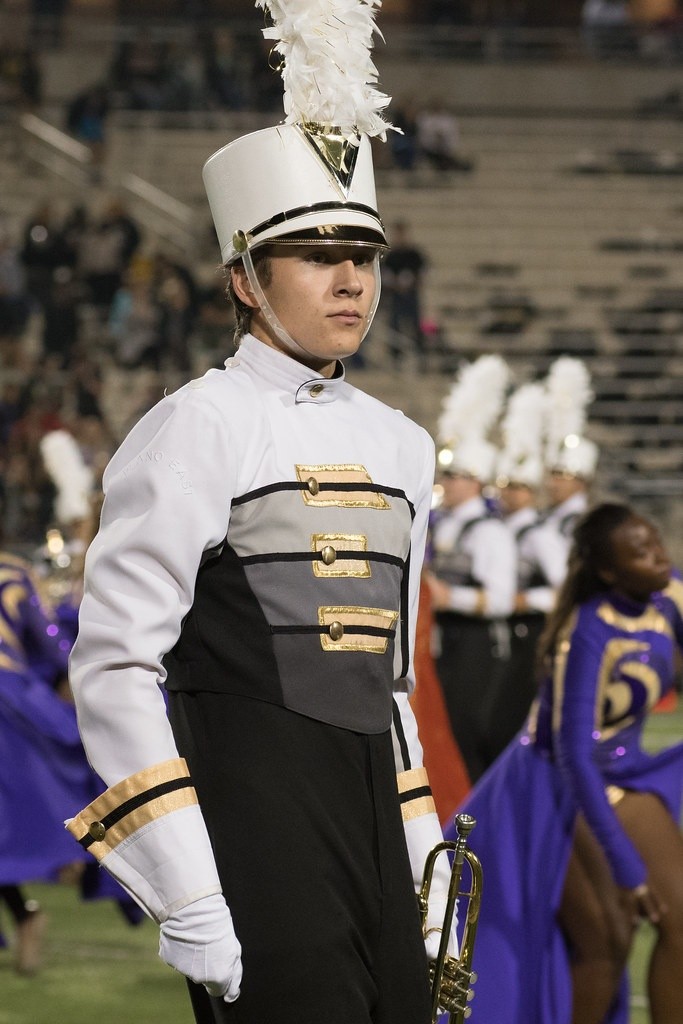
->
[16,913,48,975]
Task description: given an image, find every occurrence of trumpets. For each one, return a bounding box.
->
[417,811,485,1024]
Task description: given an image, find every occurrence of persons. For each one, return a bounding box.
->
[0,195,239,975]
[379,217,428,374]
[444,501,683,1024]
[64,121,460,1024]
[422,430,599,785]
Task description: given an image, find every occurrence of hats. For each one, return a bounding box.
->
[435,355,601,491]
[202,0,404,267]
[40,430,97,526]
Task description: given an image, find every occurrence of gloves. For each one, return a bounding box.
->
[64,757,243,1003]
[395,766,460,1015]
